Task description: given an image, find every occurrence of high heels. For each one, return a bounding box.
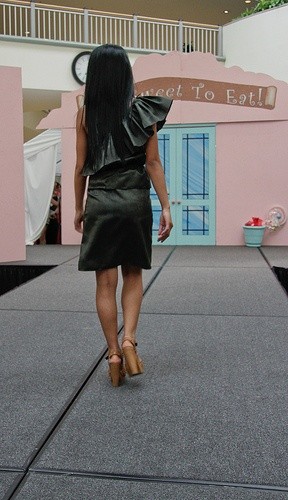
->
[105,351,125,387]
[122,337,143,377]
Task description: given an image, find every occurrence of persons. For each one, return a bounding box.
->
[72,43,175,387]
